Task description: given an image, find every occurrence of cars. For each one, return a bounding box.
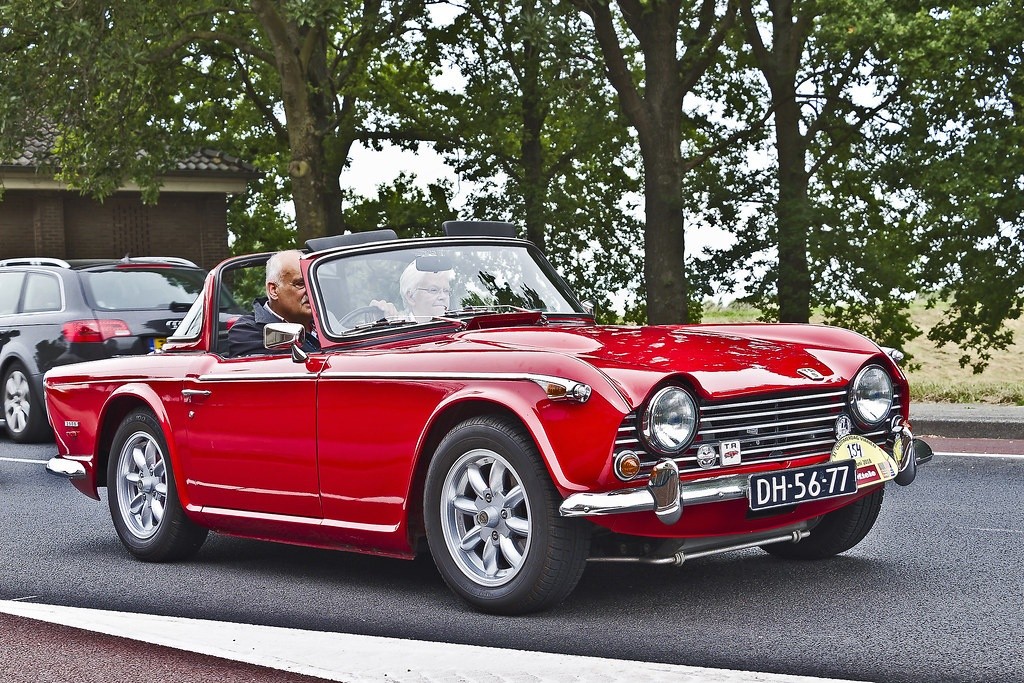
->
[47,221,935,616]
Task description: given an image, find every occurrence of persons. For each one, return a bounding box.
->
[229,248,398,359]
[398,255,456,324]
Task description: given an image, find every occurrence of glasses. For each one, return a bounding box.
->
[415,287,454,297]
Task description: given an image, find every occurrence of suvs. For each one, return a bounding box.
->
[1,255,253,445]
